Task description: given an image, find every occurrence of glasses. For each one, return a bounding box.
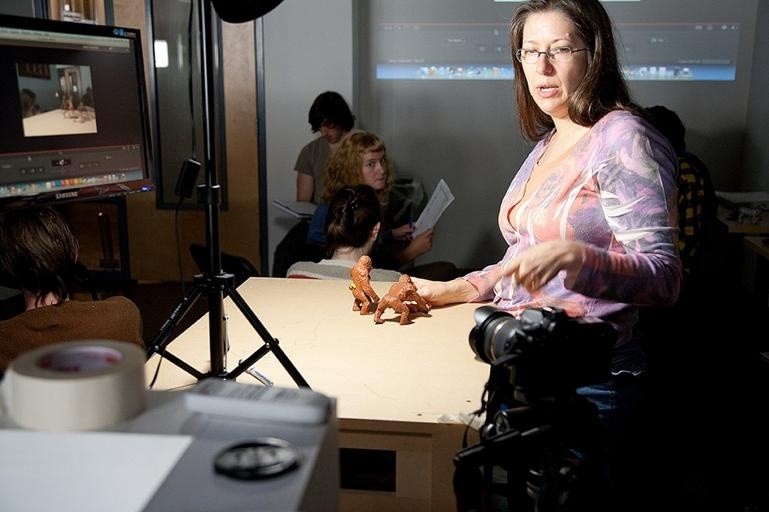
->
[515,46,587,63]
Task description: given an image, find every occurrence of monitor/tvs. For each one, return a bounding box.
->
[0,12,151,205]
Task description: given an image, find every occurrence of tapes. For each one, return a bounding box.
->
[0,337,146,433]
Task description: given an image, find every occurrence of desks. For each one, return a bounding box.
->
[139,275,498,509]
[713,189,767,289]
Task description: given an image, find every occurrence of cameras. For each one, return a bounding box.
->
[466,305,617,412]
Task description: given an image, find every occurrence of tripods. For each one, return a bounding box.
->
[144,8,318,396]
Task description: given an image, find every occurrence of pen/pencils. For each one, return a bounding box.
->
[239,358,275,387]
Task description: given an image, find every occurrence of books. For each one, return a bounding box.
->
[406,177,456,241]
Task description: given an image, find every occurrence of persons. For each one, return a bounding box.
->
[20,86,95,117]
[349,255,381,315]
[271,91,356,278]
[307,129,433,270]
[413,0,682,510]
[286,183,401,282]
[1,201,148,376]
[644,105,719,290]
[372,273,429,325]
